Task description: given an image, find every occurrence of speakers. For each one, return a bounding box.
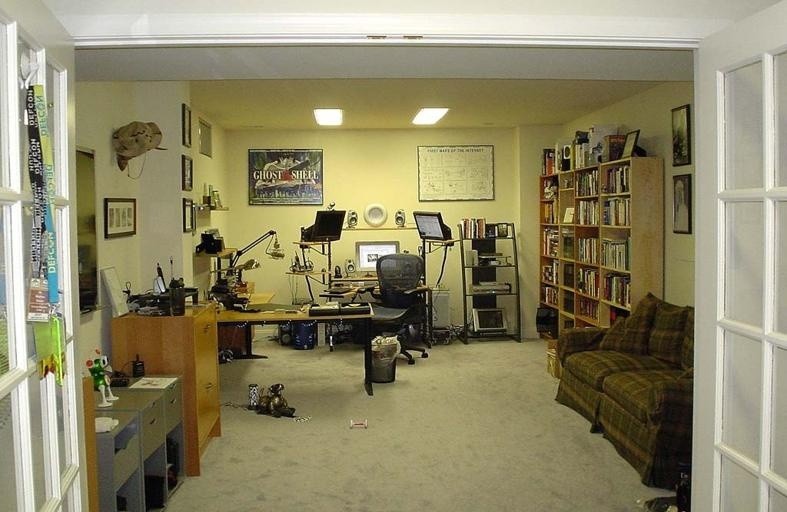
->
[348,210,358,228]
[345,258,355,277]
[395,209,405,227]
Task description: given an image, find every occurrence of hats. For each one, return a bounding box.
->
[111,121,162,158]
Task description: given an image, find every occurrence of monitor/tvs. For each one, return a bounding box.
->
[413,211,452,240]
[472,309,507,332]
[301,210,346,241]
[355,241,400,277]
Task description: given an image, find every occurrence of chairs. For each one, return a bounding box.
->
[358,253,433,365]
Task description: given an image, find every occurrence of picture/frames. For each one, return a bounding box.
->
[673,174,693,234]
[621,129,641,159]
[671,104,692,167]
[248,147,325,207]
[103,197,137,239]
[181,103,195,232]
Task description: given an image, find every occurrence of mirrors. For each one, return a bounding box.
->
[75,144,99,316]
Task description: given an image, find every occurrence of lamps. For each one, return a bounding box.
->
[227,230,286,276]
[236,259,263,285]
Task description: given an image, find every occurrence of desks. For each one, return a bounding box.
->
[216,292,375,397]
[325,276,428,343]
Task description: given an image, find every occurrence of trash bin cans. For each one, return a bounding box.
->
[369,343,397,383]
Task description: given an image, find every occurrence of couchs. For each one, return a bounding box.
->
[555,326,694,490]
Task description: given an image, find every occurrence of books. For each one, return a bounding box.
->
[536,129,631,339]
[310,302,369,316]
[463,216,486,240]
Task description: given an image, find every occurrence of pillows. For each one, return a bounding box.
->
[600,292,695,368]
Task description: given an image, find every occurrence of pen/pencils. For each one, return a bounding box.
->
[170,256,173,283]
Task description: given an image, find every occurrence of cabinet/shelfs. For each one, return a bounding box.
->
[95,373,187,512]
[111,300,222,478]
[457,222,523,345]
[538,156,665,340]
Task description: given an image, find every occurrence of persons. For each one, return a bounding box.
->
[674,179,688,231]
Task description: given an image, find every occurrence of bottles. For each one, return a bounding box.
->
[169,280,186,316]
[248,384,259,409]
